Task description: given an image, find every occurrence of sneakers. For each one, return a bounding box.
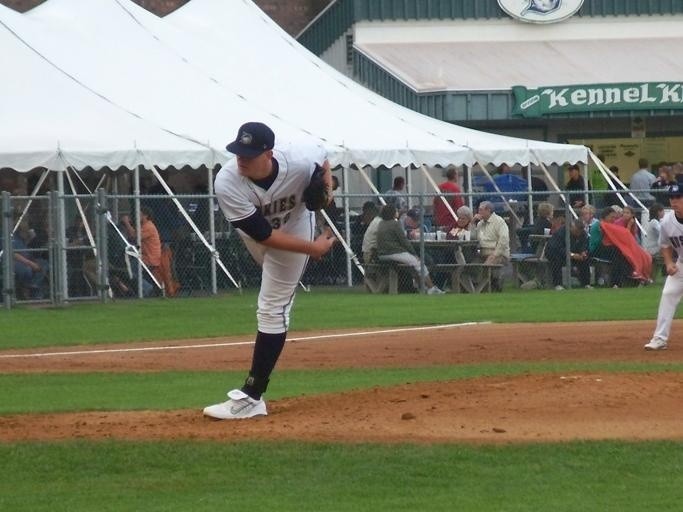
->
[521,279,621,291]
[426,285,447,295]
[644,337,668,353]
[202,387,269,421]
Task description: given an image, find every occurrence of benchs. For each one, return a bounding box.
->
[0,212,666,294]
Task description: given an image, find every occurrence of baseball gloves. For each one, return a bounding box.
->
[305,162,330,211]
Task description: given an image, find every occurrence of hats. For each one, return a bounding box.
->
[224,121,277,158]
[667,181,683,198]
[407,207,421,222]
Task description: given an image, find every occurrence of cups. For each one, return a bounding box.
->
[28,227,37,236]
[456,230,472,241]
[189,230,230,241]
[412,230,446,240]
[64,236,84,245]
[543,228,551,236]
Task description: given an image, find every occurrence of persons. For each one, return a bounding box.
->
[201,122,335,419]
[643,184,682,350]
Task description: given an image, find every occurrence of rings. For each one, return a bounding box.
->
[670,272,672,275]
